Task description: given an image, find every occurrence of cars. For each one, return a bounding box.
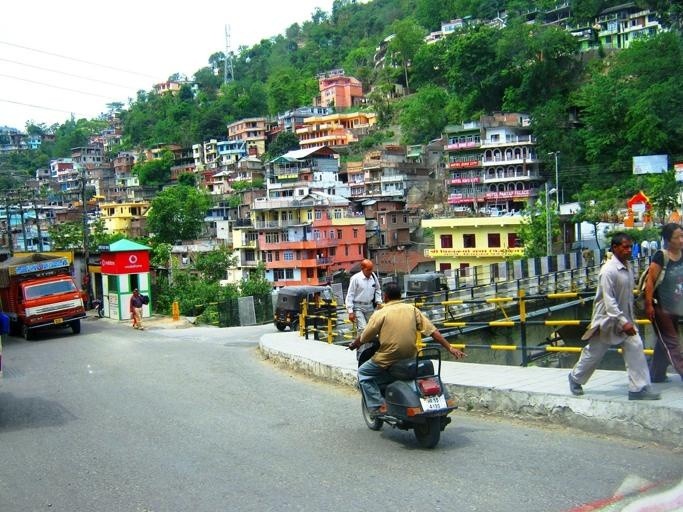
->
[453,206,539,217]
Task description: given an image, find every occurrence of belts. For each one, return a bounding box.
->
[354,302,370,305]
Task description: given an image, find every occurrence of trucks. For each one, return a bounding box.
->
[0,250,88,343]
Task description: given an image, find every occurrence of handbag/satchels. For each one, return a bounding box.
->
[371,273,377,309]
[139,293,149,305]
[635,248,669,292]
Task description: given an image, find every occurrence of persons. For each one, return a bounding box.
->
[344,258,382,362]
[567,231,662,401]
[129,288,144,330]
[644,223,683,383]
[569,204,680,270]
[347,282,468,417]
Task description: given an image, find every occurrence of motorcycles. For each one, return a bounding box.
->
[92,299,104,317]
[343,332,458,451]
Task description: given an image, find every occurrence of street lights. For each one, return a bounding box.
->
[547,150,563,207]
[641,240,658,265]
[546,187,557,256]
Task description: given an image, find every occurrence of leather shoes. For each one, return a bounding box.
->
[569,373,583,395]
[628,388,660,400]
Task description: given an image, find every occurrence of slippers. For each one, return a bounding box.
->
[368,407,387,417]
